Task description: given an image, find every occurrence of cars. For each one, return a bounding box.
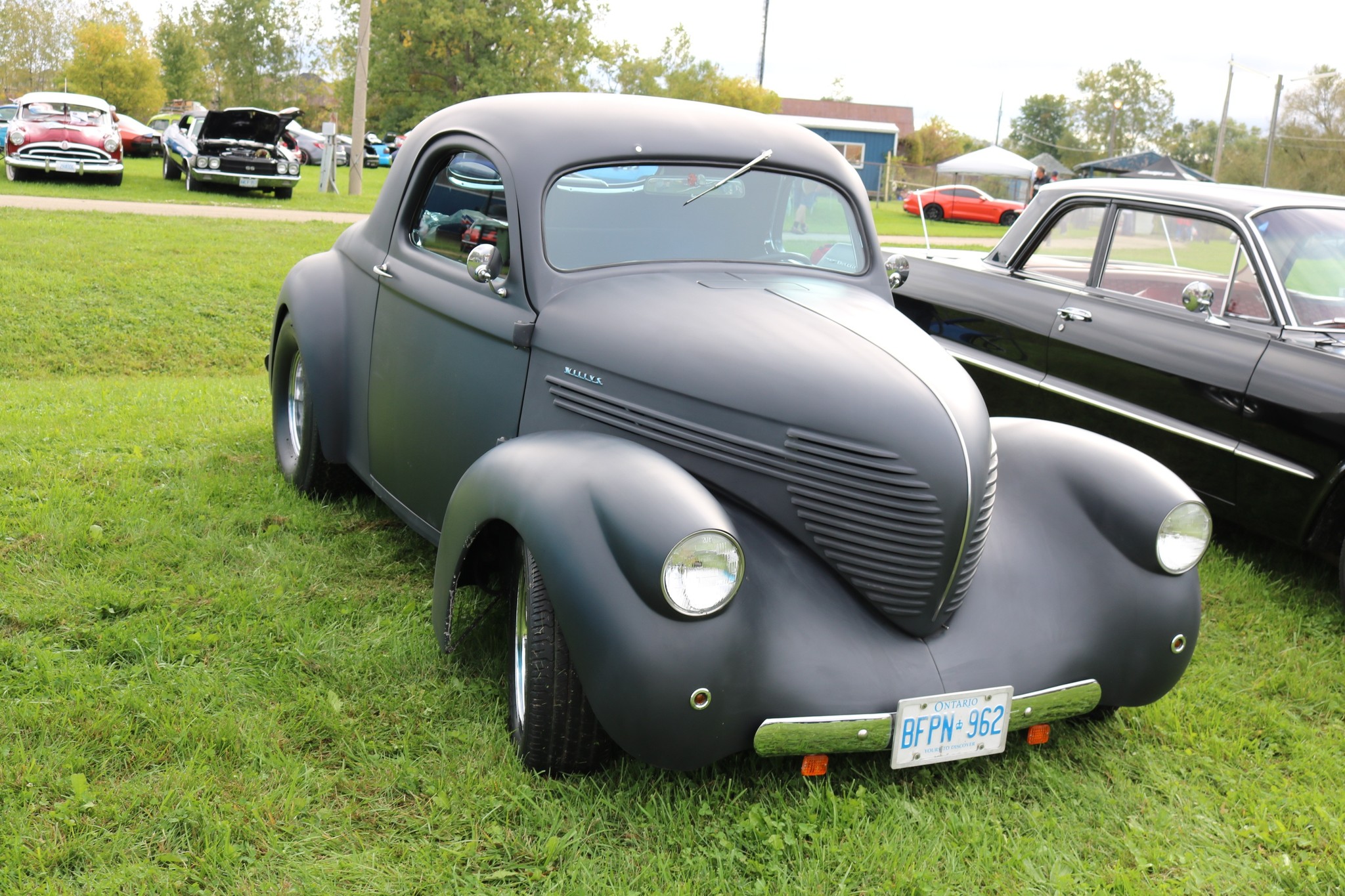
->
[320,130,410,169]
[817,172,1345,601]
[3,92,124,187]
[116,113,163,158]
[146,111,195,147]
[0,105,38,151]
[255,91,1212,787]
[283,119,325,165]
[900,182,1029,229]
[161,107,303,200]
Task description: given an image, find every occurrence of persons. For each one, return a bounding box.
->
[1026,166,1059,202]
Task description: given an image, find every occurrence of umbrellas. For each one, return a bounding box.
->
[1117,156,1201,264]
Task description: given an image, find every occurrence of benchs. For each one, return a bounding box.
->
[1027,263,1259,306]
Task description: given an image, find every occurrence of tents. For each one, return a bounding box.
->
[1073,152,1219,185]
[1008,153,1075,204]
[936,145,1040,223]
